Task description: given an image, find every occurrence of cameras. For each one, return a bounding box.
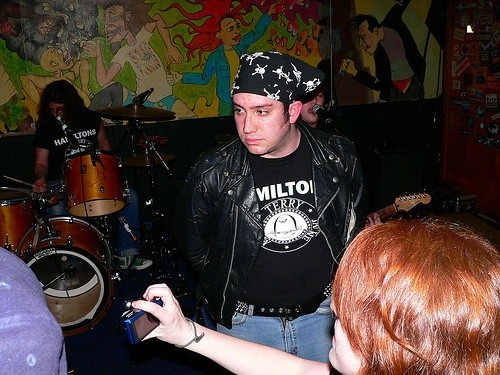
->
[120,297,163,344]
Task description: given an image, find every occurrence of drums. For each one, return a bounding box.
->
[62,148,126,215]
[0,190,33,255]
[17,216,115,338]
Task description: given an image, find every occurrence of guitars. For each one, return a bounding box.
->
[352,190,432,236]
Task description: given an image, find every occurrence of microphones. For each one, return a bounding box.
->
[67,264,75,278]
[133,88,153,104]
[55,115,80,146]
[313,104,332,125]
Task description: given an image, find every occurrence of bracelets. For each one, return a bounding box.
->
[175,317,204,348]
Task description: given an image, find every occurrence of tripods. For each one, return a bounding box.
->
[123,121,210,307]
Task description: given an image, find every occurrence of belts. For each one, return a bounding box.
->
[235,280,334,320]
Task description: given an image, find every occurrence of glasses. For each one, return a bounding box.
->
[329,303,339,321]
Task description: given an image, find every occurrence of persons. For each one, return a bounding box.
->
[175,52,362,375]
[299,82,381,225]
[32,79,153,270]
[132,220,500,375]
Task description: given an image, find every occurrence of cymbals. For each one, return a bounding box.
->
[95,106,175,121]
[122,156,140,166]
